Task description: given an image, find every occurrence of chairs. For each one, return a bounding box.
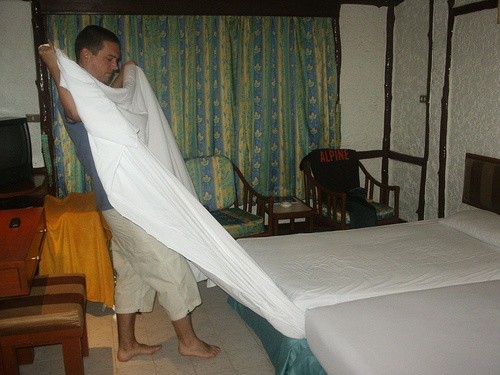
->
[181,154,275,238]
[300,148,401,228]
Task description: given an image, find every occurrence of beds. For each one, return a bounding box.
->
[233,149,499,375]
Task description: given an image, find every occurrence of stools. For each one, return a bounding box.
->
[1,272,88,375]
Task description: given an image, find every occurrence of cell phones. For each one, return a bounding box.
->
[9,217,20,228]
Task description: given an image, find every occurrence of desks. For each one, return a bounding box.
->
[0,207,49,300]
[262,193,316,234]
[0,168,51,204]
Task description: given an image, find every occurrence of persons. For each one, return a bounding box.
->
[38,24,222,361]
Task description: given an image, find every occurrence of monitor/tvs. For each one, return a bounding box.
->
[0,116,36,194]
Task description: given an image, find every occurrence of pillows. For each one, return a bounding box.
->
[437,208,499,246]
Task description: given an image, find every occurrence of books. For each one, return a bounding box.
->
[263,196,298,205]
